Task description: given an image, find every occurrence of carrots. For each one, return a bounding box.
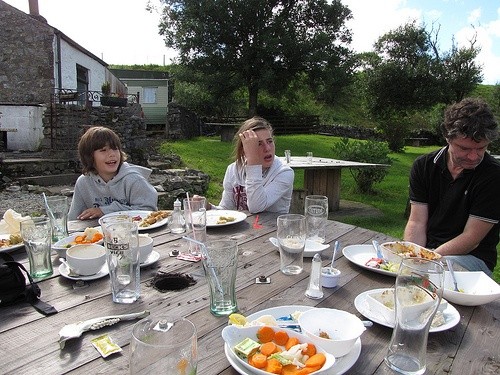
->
[70,234,103,244]
[249,327,326,375]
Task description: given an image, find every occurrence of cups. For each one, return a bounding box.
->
[385,257,445,375]
[184,197,206,254]
[129,315,198,375]
[20,196,69,279]
[277,195,328,275]
[200,240,239,316]
[307,152,312,164]
[285,150,290,163]
[102,215,140,303]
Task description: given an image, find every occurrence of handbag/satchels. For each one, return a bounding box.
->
[0,250,58,316]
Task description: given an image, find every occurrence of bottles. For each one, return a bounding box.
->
[169,199,185,234]
[305,253,324,300]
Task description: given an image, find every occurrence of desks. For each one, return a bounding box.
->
[275,157,392,211]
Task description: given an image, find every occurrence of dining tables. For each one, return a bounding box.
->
[0,212,500,375]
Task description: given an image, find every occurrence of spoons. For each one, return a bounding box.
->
[59,257,79,277]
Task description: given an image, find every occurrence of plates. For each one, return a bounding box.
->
[224,305,361,375]
[0,235,24,254]
[98,210,247,230]
[269,237,330,257]
[59,250,160,280]
[342,245,424,278]
[354,288,460,332]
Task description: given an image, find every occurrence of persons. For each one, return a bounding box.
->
[192,117,294,214]
[66,127,159,221]
[402,97,500,275]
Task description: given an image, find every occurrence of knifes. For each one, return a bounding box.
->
[372,240,386,264]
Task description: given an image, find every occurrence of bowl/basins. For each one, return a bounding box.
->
[381,242,442,265]
[367,291,448,324]
[428,270,500,306]
[320,267,341,288]
[299,308,367,358]
[222,325,335,375]
[52,233,153,276]
